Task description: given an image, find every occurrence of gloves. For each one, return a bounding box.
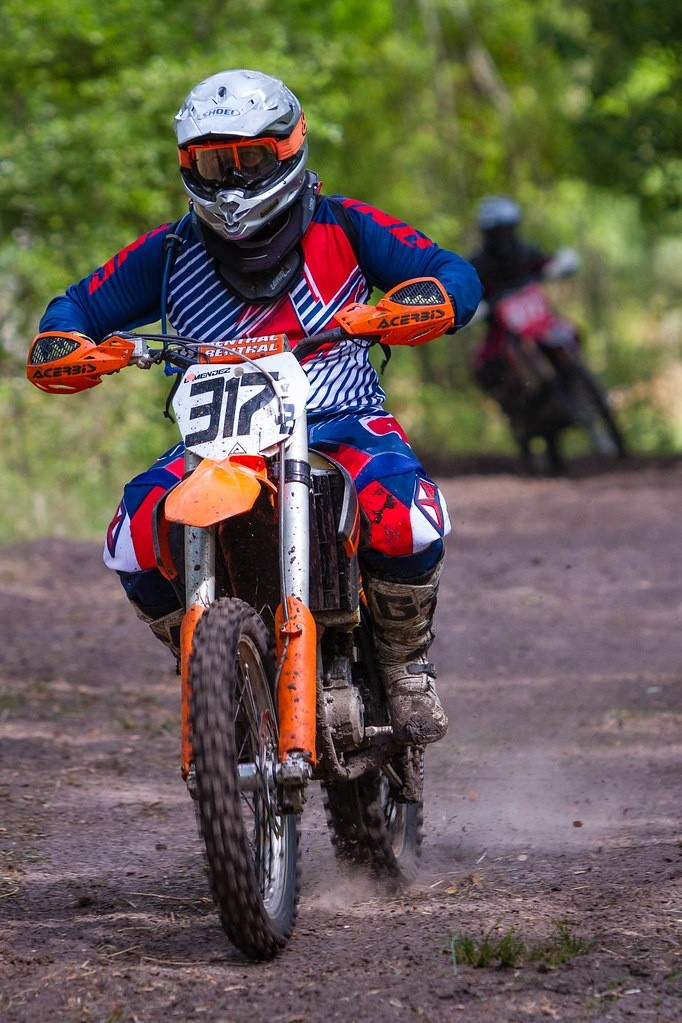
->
[31,331,96,363]
[402,294,444,305]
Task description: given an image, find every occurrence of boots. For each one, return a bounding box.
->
[362,547,449,746]
[125,600,185,676]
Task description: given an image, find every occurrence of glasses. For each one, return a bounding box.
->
[195,146,266,183]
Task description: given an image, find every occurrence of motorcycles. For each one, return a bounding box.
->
[24,275,455,967]
[457,248,630,478]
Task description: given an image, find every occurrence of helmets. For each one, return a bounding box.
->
[478,197,521,229]
[172,69,308,240]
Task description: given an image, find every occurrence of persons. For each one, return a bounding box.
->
[464,196,581,440]
[26,70,484,747]
